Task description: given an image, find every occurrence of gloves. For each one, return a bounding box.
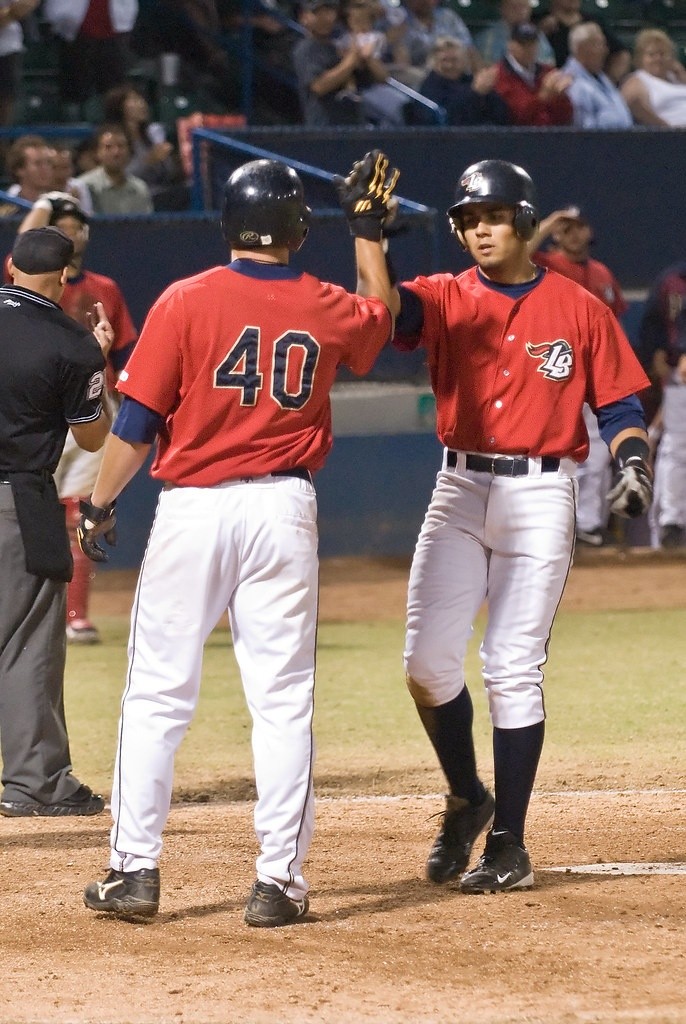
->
[604,456,653,520]
[344,160,399,255]
[76,492,118,564]
[331,149,401,242]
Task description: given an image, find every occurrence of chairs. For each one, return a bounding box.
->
[445,0,686,68]
[0,0,224,126]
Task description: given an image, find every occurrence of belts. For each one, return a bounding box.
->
[270,464,311,484]
[441,448,560,477]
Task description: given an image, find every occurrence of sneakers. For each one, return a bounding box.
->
[459,826,534,894]
[427,782,495,882]
[0,782,105,817]
[244,879,309,927]
[82,867,160,917]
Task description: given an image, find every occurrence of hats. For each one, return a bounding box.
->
[555,204,587,226]
[511,21,538,43]
[13,225,76,274]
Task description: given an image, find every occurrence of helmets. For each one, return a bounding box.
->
[219,158,311,250]
[48,192,89,226]
[445,159,536,252]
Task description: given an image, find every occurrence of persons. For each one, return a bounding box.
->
[562,23,635,126]
[477,0,557,67]
[636,261,686,550]
[110,86,191,210]
[345,155,650,892]
[497,23,576,126]
[0,124,155,221]
[416,37,512,126]
[528,205,626,547]
[217,0,484,126]
[76,150,401,924]
[544,0,632,83]
[0,0,241,124]
[0,228,113,815]
[8,197,139,641]
[621,31,686,126]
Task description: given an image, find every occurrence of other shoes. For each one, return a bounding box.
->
[66,618,99,642]
